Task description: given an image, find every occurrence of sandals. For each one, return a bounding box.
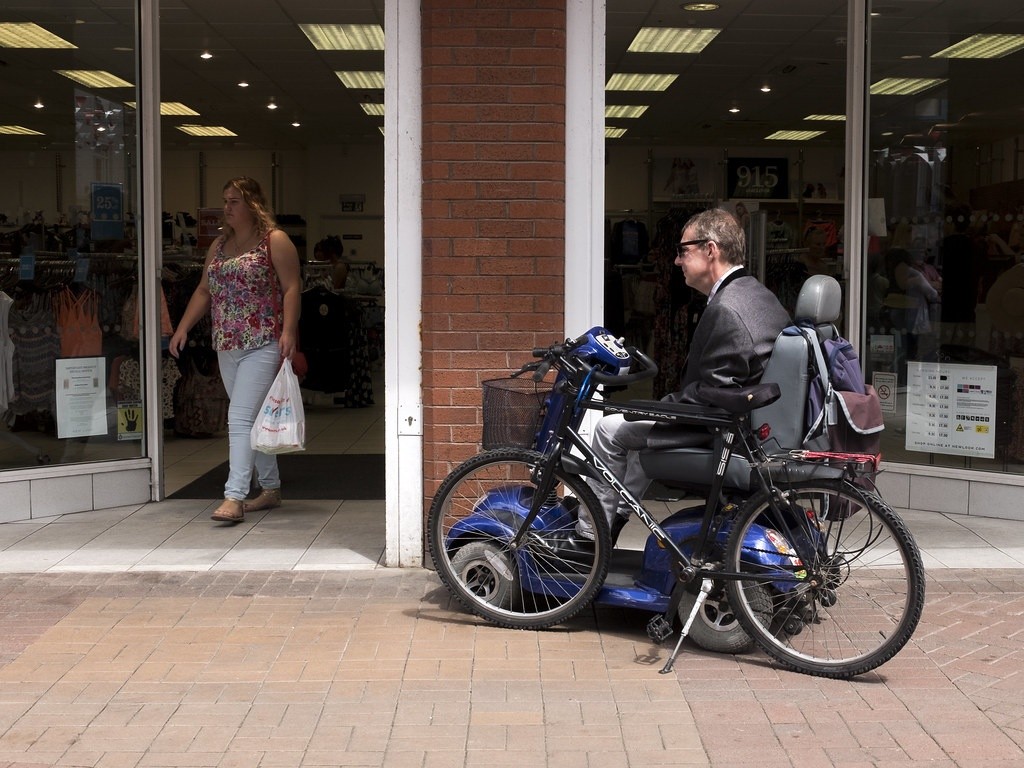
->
[243,490,281,512]
[211,498,246,521]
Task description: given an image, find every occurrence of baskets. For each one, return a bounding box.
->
[481,376,555,454]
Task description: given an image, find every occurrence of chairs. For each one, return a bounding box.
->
[640,274,841,491]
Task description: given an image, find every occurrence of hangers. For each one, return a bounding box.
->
[623,194,717,228]
[766,209,835,230]
[7,254,384,299]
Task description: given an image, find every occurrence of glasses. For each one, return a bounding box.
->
[676,240,707,258]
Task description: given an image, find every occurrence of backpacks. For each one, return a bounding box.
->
[804,321,866,440]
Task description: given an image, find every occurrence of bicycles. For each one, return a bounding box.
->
[427,335,927,674]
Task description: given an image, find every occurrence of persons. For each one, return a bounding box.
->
[732,202,755,237]
[167,176,303,523]
[545,202,800,576]
[777,157,1023,442]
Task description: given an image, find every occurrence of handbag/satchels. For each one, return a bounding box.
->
[794,321,884,522]
[251,357,306,455]
[283,352,308,385]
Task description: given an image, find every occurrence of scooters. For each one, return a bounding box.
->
[439,273,842,654]
